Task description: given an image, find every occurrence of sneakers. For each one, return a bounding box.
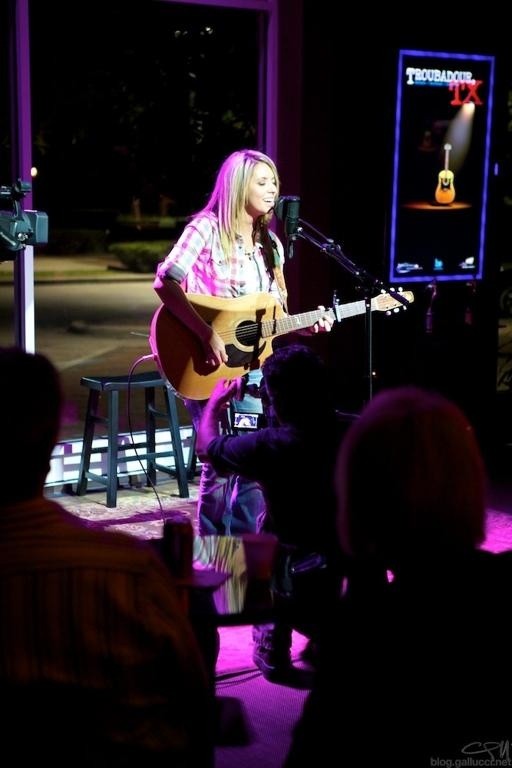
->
[252,644,289,676]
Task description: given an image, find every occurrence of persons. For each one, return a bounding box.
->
[153,149,336,536]
[0,344,512,768]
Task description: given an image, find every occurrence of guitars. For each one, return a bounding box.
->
[147,286,416,401]
[435,143,456,204]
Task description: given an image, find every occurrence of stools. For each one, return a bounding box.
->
[76,371,189,507]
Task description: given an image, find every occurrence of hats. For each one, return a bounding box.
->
[337,385,487,552]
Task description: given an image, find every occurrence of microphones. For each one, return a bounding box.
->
[275,197,334,244]
[284,196,300,260]
